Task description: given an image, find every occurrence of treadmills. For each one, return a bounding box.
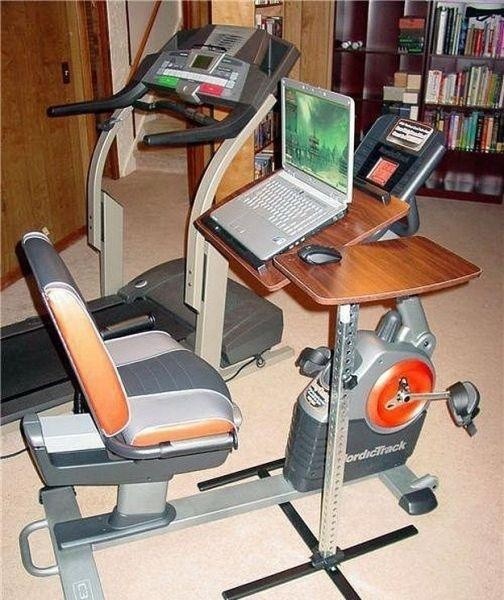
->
[1,26,300,424]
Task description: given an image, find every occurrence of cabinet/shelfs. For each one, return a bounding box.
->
[180,0,335,208]
[331,0,503,205]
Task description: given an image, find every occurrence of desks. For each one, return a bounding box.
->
[190,169,484,599]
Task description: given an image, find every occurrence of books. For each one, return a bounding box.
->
[382,4,504,153]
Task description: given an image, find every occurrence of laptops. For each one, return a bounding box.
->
[201,76,355,263]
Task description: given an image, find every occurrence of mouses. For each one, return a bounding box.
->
[298,244,343,266]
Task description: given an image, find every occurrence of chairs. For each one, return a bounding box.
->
[16,228,243,551]
